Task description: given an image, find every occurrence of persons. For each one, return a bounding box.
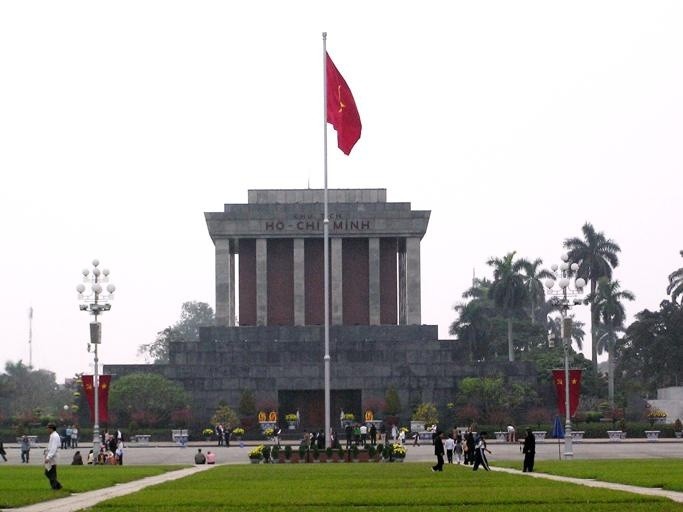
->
[21,436,31,463]
[303,422,492,474]
[507,424,515,442]
[522,429,535,472]
[43,423,63,490]
[60,424,124,465]
[215,424,224,446]
[223,425,231,447]
[206,451,215,464]
[194,449,206,464]
[0,438,7,462]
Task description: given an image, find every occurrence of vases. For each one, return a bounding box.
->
[250,458,258,463]
[395,457,403,462]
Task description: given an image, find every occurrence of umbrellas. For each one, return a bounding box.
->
[551,415,565,460]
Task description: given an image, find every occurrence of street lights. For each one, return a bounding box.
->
[75,257,116,463]
[544,252,587,461]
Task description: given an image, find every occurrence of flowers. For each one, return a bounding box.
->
[248,449,261,459]
[392,448,407,458]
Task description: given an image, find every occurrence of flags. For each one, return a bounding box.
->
[324,51,362,156]
